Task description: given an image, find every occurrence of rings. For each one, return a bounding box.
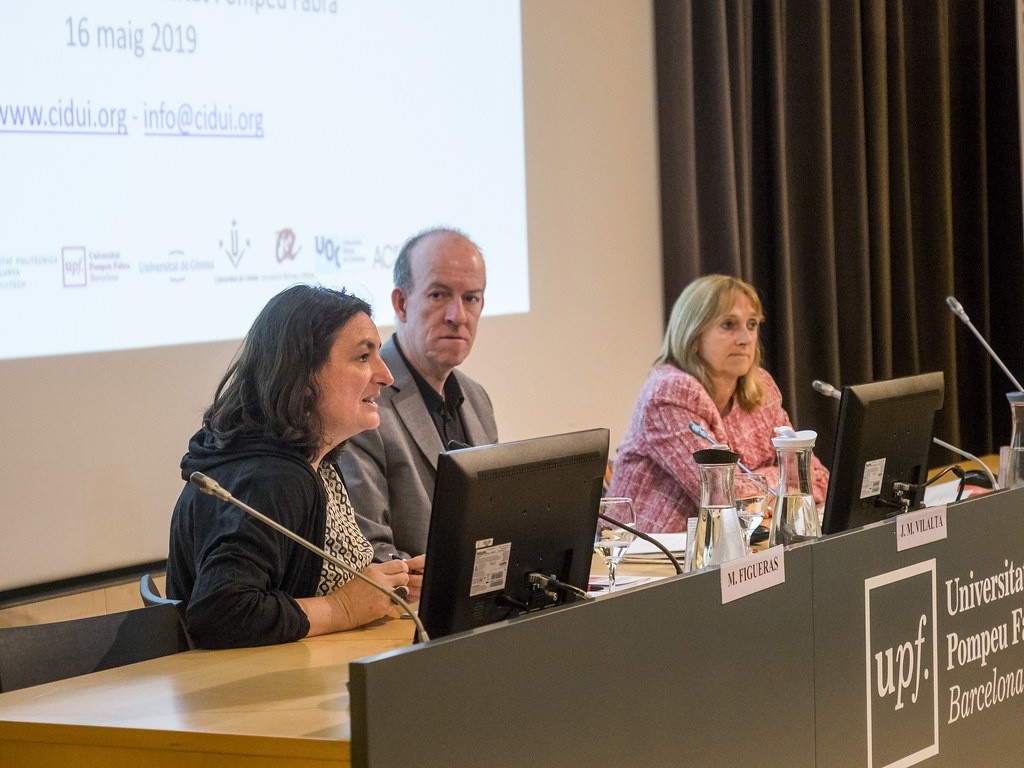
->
[391,586,408,605]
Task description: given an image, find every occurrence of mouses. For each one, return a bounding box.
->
[965,470,998,489]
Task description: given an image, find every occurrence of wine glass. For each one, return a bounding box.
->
[593,497,637,594]
[733,473,768,558]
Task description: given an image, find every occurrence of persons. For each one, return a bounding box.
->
[336,227,498,562]
[598,273,829,533]
[164,284,427,649]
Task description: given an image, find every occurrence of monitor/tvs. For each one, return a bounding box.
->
[822,371,944,534]
[412,427,611,646]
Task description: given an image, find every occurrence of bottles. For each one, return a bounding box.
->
[768,426,822,548]
[681,448,751,575]
[1004,392,1024,489]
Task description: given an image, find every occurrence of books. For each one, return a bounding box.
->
[623,533,686,565]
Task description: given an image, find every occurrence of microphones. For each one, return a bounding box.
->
[689,421,777,497]
[448,439,683,575]
[812,379,1000,491]
[190,472,430,643]
[945,296,1024,393]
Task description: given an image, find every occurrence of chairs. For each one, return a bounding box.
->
[137,573,198,652]
[1,604,174,694]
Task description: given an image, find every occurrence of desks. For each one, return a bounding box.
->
[1,456,1007,768]
